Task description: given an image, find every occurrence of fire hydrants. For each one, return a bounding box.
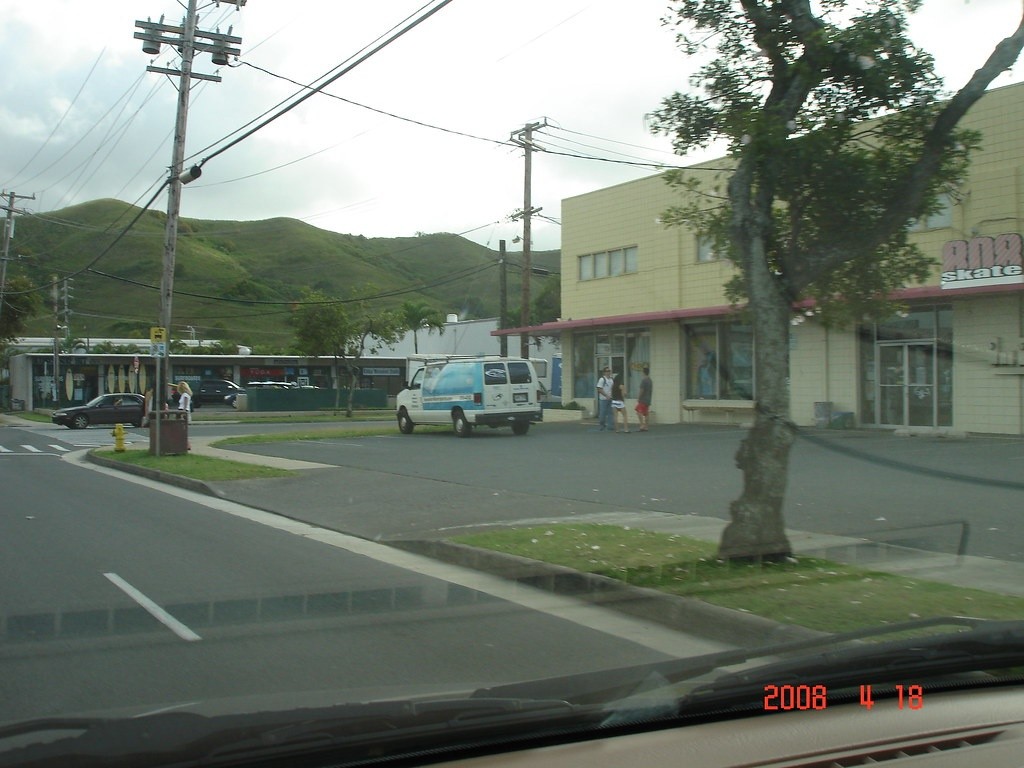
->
[110,423,129,452]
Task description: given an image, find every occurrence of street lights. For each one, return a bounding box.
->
[187,326,195,340]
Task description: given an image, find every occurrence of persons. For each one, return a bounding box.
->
[114,398,124,407]
[176,380,193,451]
[634,368,652,432]
[597,367,632,433]
[145,387,169,422]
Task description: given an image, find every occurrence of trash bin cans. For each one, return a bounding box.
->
[813,401,832,429]
[829,411,854,430]
[149,410,189,455]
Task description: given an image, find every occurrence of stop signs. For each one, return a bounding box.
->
[133,357,140,369]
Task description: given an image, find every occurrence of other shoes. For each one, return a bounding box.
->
[187,446,192,450]
[600,423,605,431]
[624,430,632,433]
[616,430,622,433]
[636,428,643,432]
[608,426,615,430]
[640,426,648,430]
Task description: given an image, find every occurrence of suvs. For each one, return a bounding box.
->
[171,376,240,408]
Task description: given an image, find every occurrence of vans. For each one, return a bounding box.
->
[394,354,544,436]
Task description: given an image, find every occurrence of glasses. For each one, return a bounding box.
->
[604,370,611,372]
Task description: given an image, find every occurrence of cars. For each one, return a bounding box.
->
[51,392,169,430]
[224,380,320,409]
